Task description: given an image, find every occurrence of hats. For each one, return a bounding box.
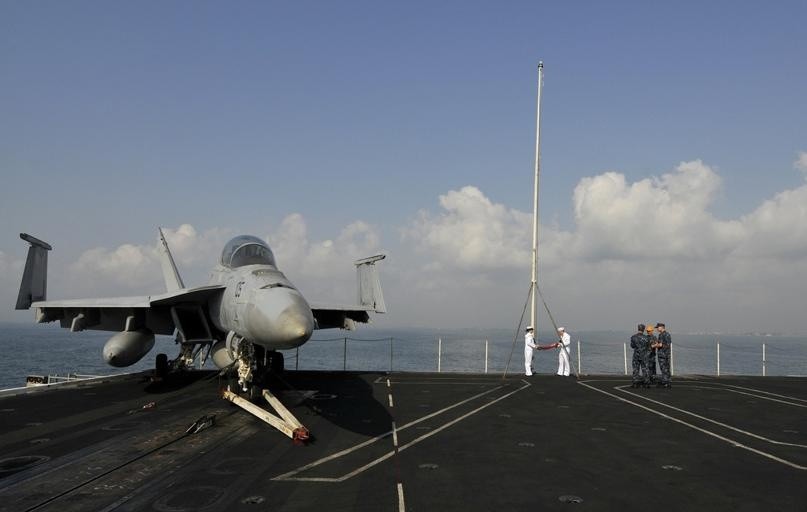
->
[647,325,655,333]
[525,326,535,330]
[654,322,666,329]
[557,326,566,333]
[638,324,645,331]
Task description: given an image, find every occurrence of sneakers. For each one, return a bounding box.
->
[631,376,671,389]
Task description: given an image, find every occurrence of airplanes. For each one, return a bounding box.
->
[10,224,393,400]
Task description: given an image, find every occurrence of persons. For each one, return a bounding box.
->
[523,325,539,377]
[650,321,672,387]
[629,323,652,389]
[644,323,658,385]
[555,325,571,377]
